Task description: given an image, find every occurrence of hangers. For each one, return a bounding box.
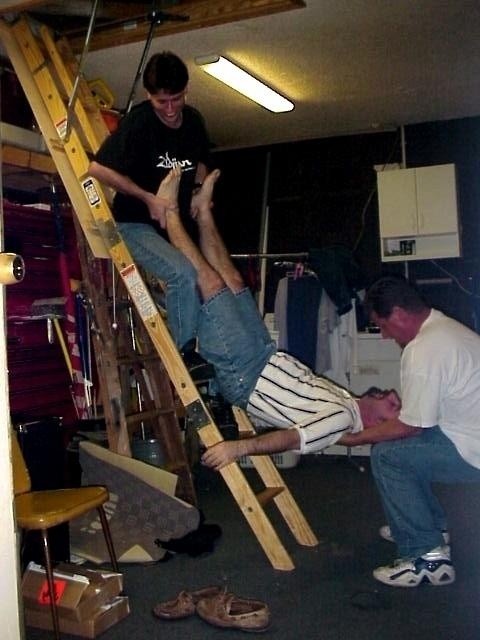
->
[286,256,318,278]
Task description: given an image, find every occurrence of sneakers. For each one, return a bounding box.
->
[378,524,450,545]
[372,544,455,588]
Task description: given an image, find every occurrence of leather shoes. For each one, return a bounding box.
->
[184,350,209,377]
[152,585,222,620]
[196,585,274,633]
[213,404,238,431]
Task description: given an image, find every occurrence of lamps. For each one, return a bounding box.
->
[196,53,294,114]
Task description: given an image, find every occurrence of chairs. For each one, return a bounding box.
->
[10,424,119,640]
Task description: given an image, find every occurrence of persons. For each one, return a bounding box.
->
[334,275,480,588]
[84,52,213,376]
[153,165,423,471]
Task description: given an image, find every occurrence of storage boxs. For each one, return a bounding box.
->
[25,597,131,639]
[21,561,124,623]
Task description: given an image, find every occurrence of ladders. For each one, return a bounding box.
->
[0,17,319,571]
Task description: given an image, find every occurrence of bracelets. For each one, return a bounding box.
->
[191,181,202,190]
